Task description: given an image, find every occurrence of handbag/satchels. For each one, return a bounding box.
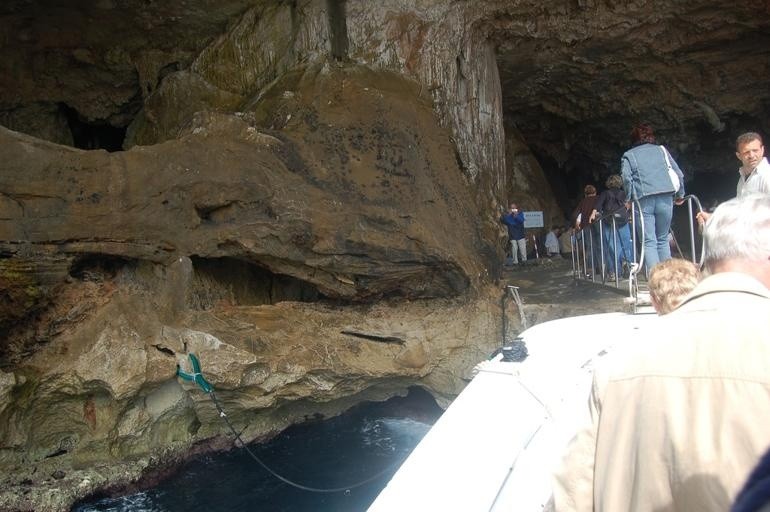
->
[668,167,681,195]
[606,207,627,230]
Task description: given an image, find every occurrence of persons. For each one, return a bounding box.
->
[553,196,770,512]
